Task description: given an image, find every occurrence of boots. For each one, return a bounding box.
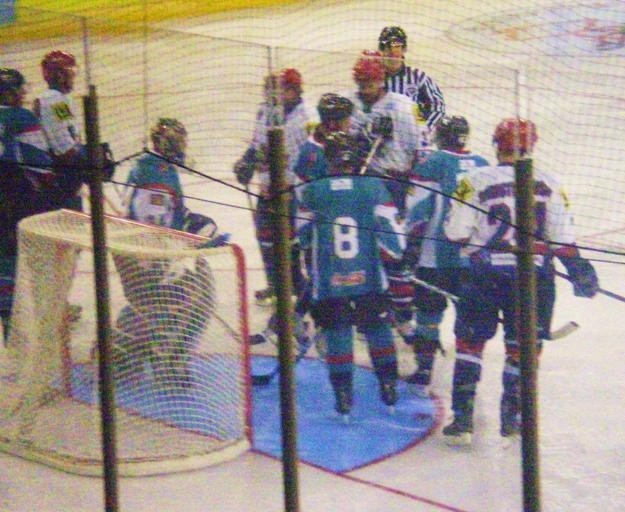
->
[333,321,432,414]
[443,405,474,436]
[501,399,523,437]
[263,27,407,169]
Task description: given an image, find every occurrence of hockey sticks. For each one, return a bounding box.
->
[388,310,414,346]
[252,342,307,386]
[211,313,265,346]
[437,336,455,359]
[409,275,579,340]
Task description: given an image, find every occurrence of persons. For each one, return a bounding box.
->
[229,27,598,435]
[85,118,218,386]
[0,69,71,341]
[30,50,113,321]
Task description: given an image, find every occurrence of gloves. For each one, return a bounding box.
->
[233,148,259,185]
[567,259,599,298]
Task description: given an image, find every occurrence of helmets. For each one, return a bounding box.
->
[435,114,469,147]
[0,68,25,106]
[150,117,187,165]
[491,118,538,153]
[41,49,76,94]
